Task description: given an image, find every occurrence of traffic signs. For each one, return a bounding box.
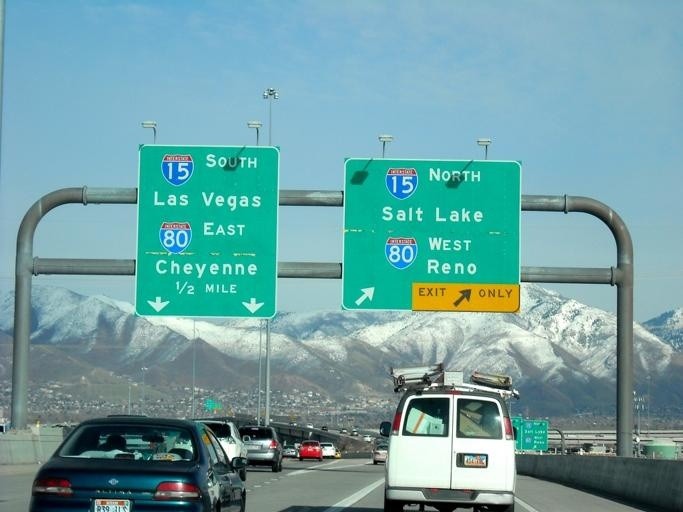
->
[494,416,521,451]
[522,419,547,451]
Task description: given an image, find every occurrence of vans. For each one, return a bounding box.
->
[304,423,312,429]
[375,360,522,511]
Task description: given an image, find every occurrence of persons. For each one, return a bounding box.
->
[107,433,129,449]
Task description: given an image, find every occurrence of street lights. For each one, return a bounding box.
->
[632,388,645,457]
[260,86,277,426]
[645,373,651,430]
[261,308,291,425]
[140,367,150,414]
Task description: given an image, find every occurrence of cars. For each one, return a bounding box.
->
[287,421,297,426]
[339,428,347,434]
[320,426,328,432]
[283,440,341,461]
[362,434,372,443]
[371,444,387,464]
[226,413,274,423]
[350,430,358,436]
[24,413,249,512]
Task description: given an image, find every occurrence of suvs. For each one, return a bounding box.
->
[236,425,284,473]
[188,418,246,482]
